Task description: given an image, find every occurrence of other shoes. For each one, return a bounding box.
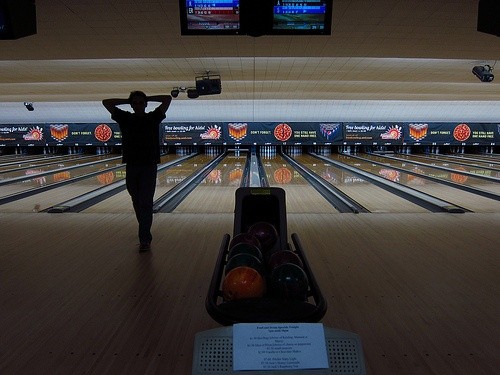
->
[140,241,150,251]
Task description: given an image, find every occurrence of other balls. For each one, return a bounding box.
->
[225,267,265,298]
[228,243,264,262]
[226,253,264,276]
[272,264,309,301]
[249,221,278,250]
[272,251,303,268]
[229,233,261,248]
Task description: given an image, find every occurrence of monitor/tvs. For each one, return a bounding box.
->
[179,0,334,38]
[0,0,37,40]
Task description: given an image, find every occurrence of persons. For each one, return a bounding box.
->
[101,90,173,252]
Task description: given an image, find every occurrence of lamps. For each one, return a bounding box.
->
[22,102,33,111]
[170,85,199,97]
[469,63,495,81]
